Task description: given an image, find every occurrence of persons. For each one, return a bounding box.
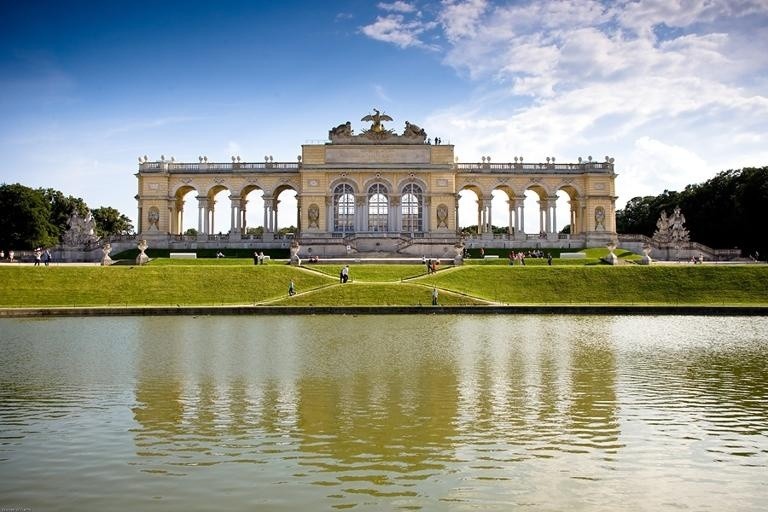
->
[422,254,426,265]
[754,250,760,259]
[43,249,51,266]
[435,255,440,265]
[431,260,436,271]
[339,268,345,284]
[427,259,432,272]
[509,248,553,266]
[426,137,432,144]
[215,250,225,258]
[435,136,438,146]
[431,287,440,306]
[259,250,265,265]
[438,137,441,145]
[289,279,297,297]
[315,255,319,263]
[254,251,259,265]
[33,247,41,265]
[0,250,5,259]
[309,256,315,263]
[691,255,697,264]
[479,247,485,259]
[699,253,704,264]
[9,250,15,263]
[343,265,349,283]
